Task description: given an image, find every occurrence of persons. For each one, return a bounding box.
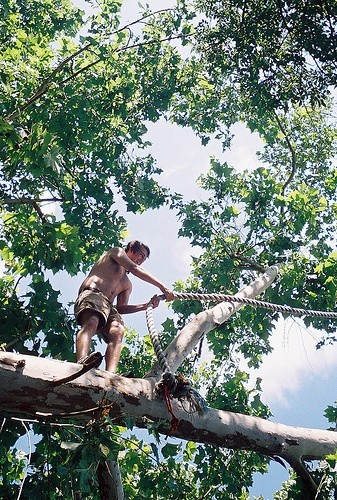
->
[73,240,174,378]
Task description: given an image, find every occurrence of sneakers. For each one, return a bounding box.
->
[77,351,103,369]
[118,371,134,379]
